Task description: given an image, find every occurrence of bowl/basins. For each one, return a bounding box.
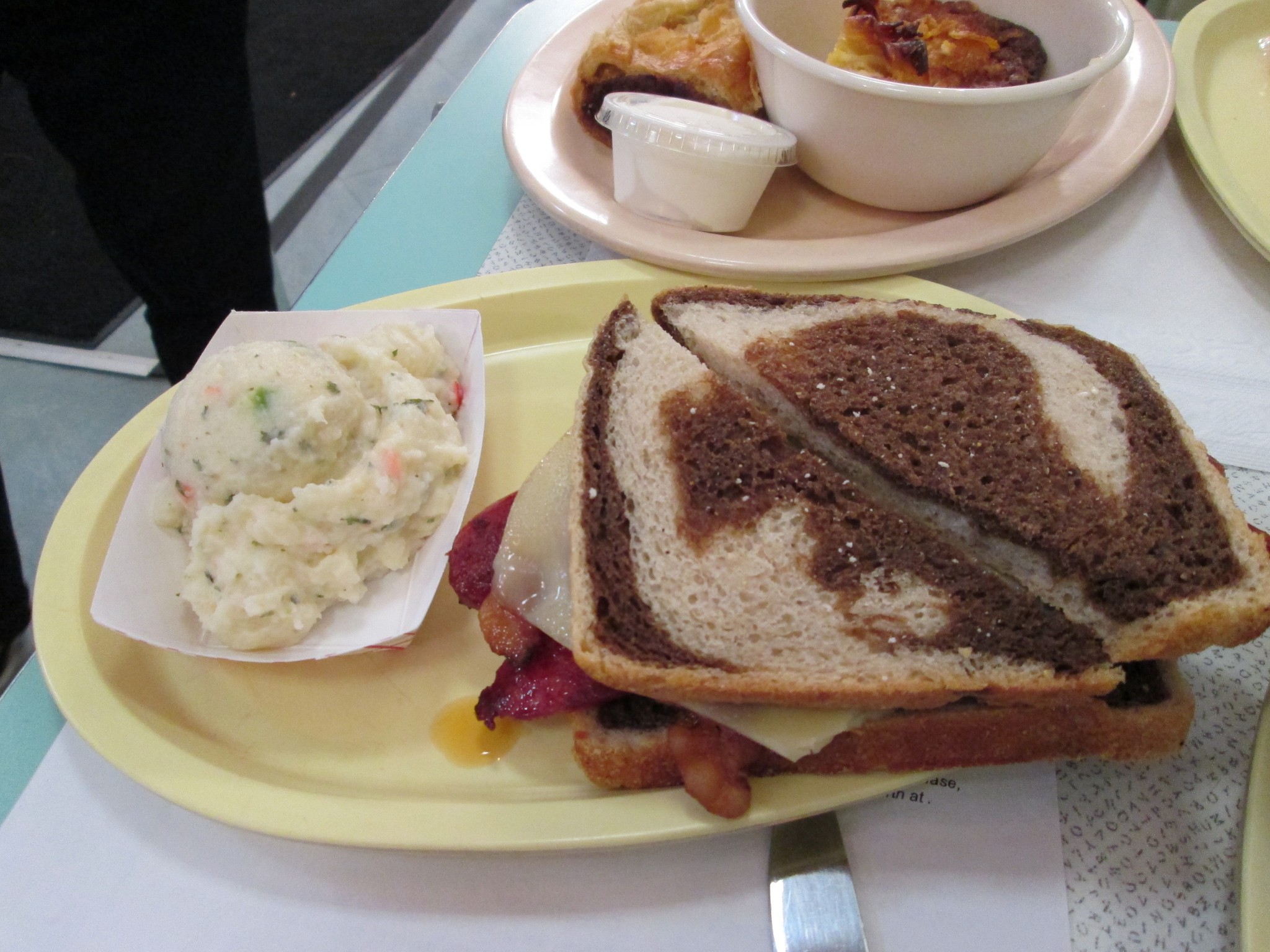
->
[593,91,798,234]
[731,1,1135,212]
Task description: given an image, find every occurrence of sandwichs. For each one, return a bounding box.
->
[444,285,1270,820]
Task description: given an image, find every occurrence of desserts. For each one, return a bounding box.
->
[825,0,1049,90]
[567,1,763,149]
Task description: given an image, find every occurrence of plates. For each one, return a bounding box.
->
[29,260,1038,856]
[501,0,1122,64]
[502,0,1174,282]
[1169,0,1269,261]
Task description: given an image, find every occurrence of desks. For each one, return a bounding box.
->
[0,0,1270,952]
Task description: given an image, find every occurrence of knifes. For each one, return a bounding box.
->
[766,813,870,952]
